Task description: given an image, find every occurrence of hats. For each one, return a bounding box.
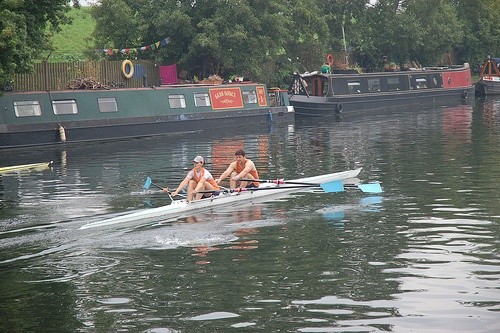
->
[192,155,204,163]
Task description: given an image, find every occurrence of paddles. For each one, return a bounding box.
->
[144,176,171,194]
[198,178,344,194]
[236,178,382,193]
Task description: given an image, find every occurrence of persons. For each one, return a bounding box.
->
[172,155,220,201]
[216,149,260,190]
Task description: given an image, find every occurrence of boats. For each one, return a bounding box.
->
[288,63,476,116]
[0,81,295,134]
[77,166,363,235]
[476,77,500,95]
[0,162,53,174]
